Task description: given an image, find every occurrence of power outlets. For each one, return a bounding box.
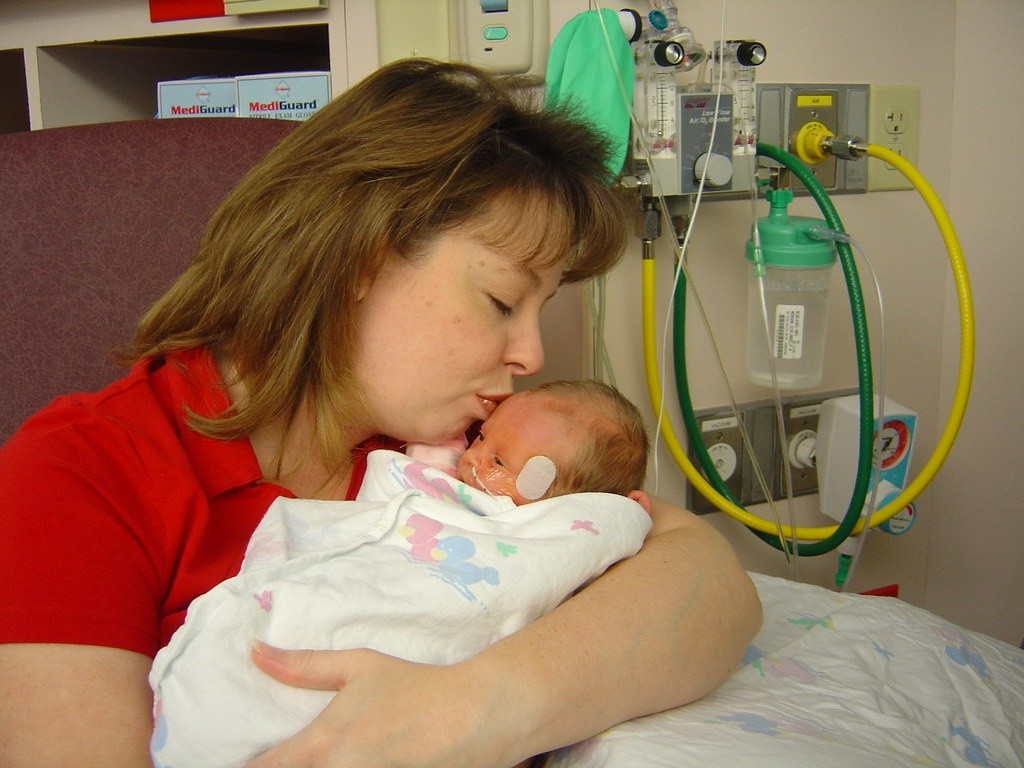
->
[867,85,921,191]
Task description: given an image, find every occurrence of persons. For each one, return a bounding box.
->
[0,56,762,768]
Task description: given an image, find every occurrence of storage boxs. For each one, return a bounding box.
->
[157,70,332,123]
